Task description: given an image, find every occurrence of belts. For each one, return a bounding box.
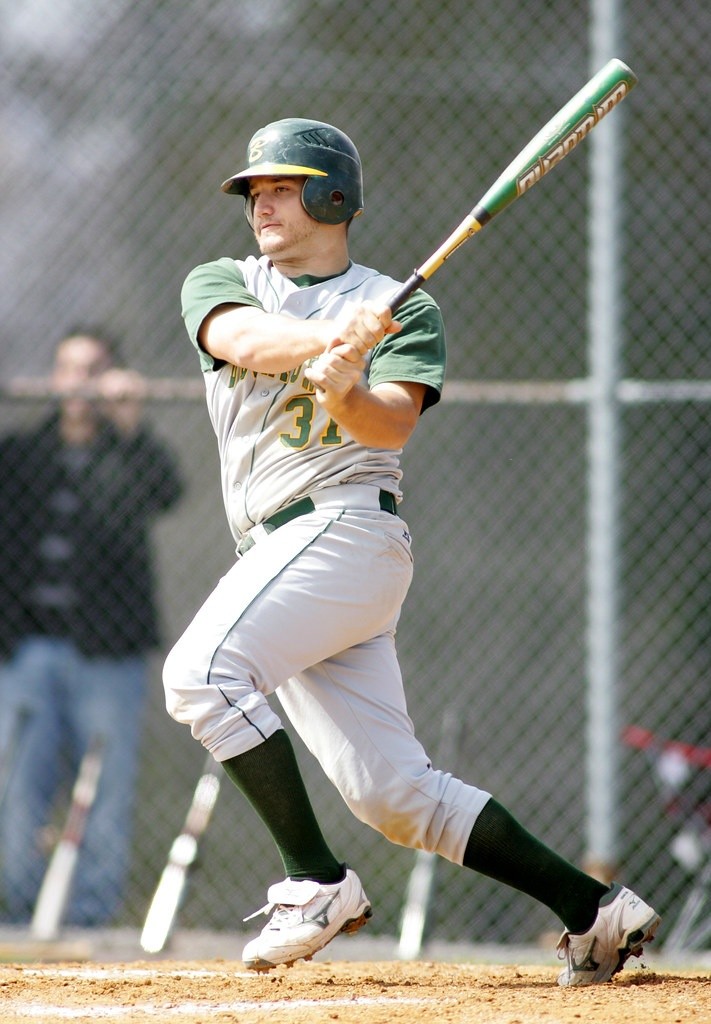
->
[238,489,397,555]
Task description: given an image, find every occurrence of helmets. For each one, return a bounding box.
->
[220,117,365,225]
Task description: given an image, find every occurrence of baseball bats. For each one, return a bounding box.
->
[311,56,640,394]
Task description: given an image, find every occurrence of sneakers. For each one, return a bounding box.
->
[555,882,661,988]
[240,863,372,973]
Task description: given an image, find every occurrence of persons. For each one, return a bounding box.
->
[0,324,183,931]
[162,117,664,989]
